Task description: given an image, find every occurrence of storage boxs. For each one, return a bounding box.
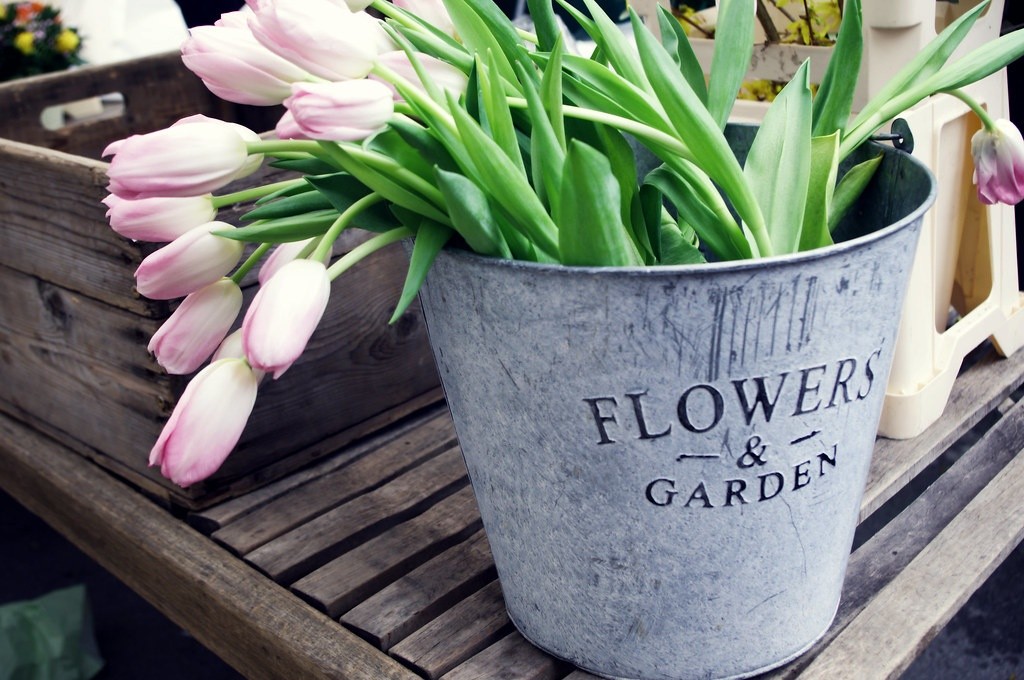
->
[0,45,439,509]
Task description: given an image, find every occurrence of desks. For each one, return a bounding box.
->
[0,342,1024,680]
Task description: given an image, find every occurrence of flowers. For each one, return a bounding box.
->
[0,0,88,83]
[103,0,1024,488]
